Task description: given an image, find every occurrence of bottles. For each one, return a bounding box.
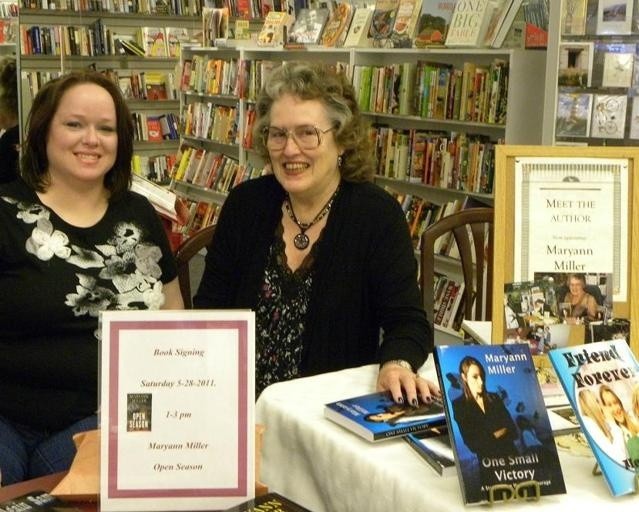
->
[536,335,545,355]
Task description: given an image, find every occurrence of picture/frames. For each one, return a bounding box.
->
[490,145,638,365]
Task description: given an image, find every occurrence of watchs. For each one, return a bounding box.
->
[379,359,413,372]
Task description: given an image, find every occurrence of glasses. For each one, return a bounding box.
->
[262,123,338,151]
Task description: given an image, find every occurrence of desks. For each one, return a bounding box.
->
[256,354,639,512]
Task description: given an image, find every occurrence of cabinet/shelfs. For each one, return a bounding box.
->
[0,0,16,55]
[171,0,559,351]
[555,0,639,149]
[16,0,204,187]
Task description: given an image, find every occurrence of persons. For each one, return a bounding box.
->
[192,58,445,408]
[451,356,546,500]
[581,382,639,476]
[515,274,628,356]
[1,69,186,492]
[363,398,443,427]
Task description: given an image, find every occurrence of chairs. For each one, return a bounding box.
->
[422,207,495,351]
[171,224,216,308]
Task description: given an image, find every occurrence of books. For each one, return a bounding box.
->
[2,1,546,338]
[548,336,638,499]
[540,383,580,436]
[322,386,446,445]
[404,426,459,478]
[432,342,568,507]
[0,489,70,512]
[225,489,311,511]
